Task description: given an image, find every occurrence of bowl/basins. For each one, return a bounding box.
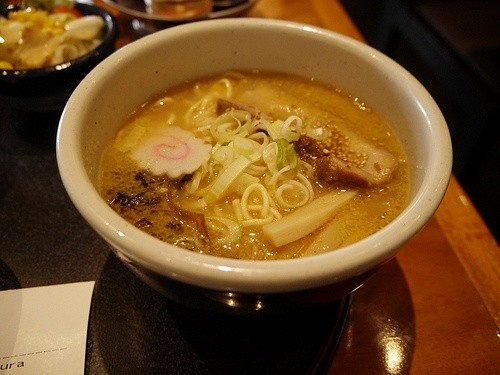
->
[53,16,453,318]
[1,0,122,120]
[84,1,256,45]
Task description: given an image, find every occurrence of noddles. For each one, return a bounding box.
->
[97,70,410,260]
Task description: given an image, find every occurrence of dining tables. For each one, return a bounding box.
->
[0,0,500,374]
